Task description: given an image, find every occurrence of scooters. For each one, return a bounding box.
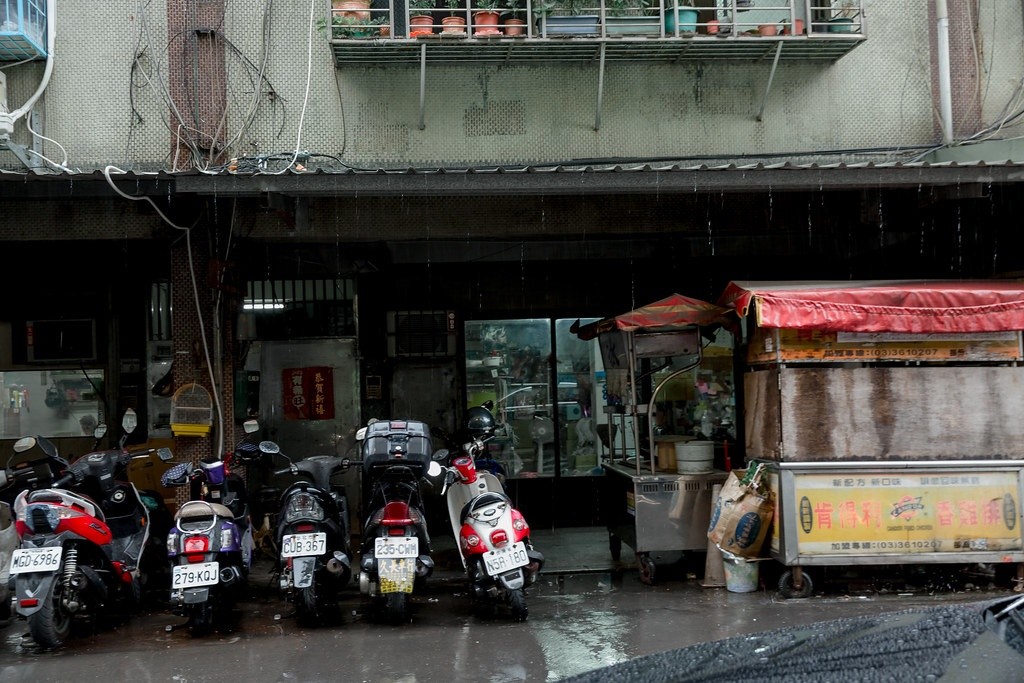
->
[156,418,260,638]
[0,407,173,651]
[257,440,365,629]
[427,401,545,622]
[355,417,432,624]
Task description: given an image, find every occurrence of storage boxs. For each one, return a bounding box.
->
[363,420,431,477]
[707,470,774,560]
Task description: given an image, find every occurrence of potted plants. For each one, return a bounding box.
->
[500,0,524,34]
[409,0,434,33]
[441,0,465,33]
[331,0,373,23]
[533,0,600,33]
[472,0,500,33]
[605,0,661,34]
[664,0,701,32]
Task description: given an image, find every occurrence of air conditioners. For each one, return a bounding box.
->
[386,311,457,357]
[146,283,175,359]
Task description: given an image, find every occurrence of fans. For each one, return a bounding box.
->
[529,418,553,474]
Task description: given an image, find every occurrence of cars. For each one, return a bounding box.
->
[491,381,585,443]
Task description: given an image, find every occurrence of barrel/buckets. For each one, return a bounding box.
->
[674,440,715,475]
[723,556,759,592]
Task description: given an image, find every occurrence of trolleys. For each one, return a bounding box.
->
[716,276,1024,599]
[572,294,741,585]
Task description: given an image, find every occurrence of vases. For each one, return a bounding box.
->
[811,20,828,32]
[758,25,776,36]
[783,18,803,34]
[829,18,854,31]
[706,20,718,33]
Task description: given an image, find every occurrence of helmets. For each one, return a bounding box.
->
[462,404,494,435]
